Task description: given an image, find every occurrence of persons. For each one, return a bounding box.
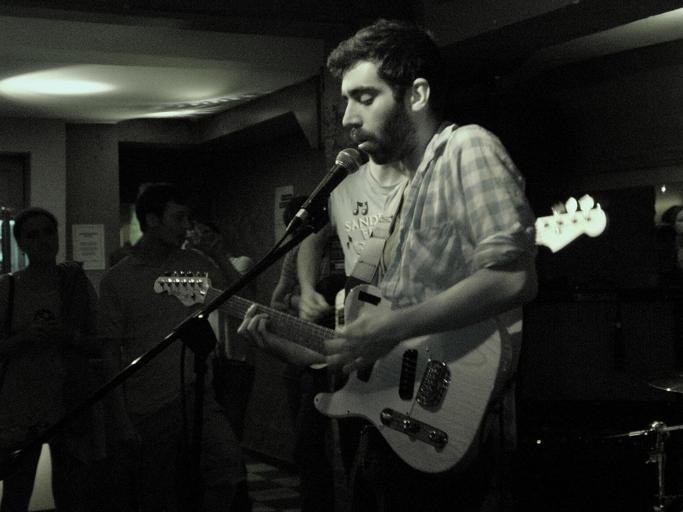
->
[104,184,241,510]
[661,205,683,273]
[0,207,101,511]
[296,220,344,331]
[200,227,254,445]
[234,20,538,511]
[270,195,331,511]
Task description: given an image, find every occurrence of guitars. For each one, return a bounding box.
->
[153,271,518,474]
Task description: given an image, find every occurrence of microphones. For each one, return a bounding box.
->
[286,147,362,234]
